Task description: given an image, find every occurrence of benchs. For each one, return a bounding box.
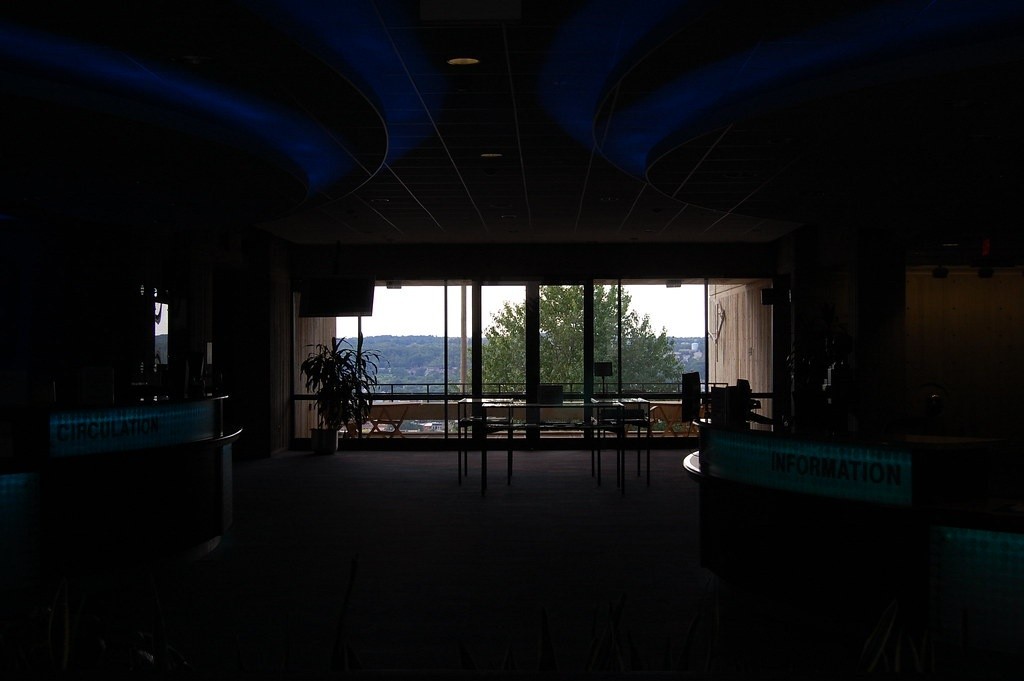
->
[343,418,369,439]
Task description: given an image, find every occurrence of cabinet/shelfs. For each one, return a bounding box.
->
[590,397,651,488]
[457,398,513,486]
[481,402,625,498]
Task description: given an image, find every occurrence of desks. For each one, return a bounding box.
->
[365,403,421,442]
[651,401,705,438]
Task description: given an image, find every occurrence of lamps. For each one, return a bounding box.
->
[664,280,682,287]
[386,280,402,288]
[595,362,612,399]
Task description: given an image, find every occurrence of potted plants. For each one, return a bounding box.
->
[299,332,390,457]
